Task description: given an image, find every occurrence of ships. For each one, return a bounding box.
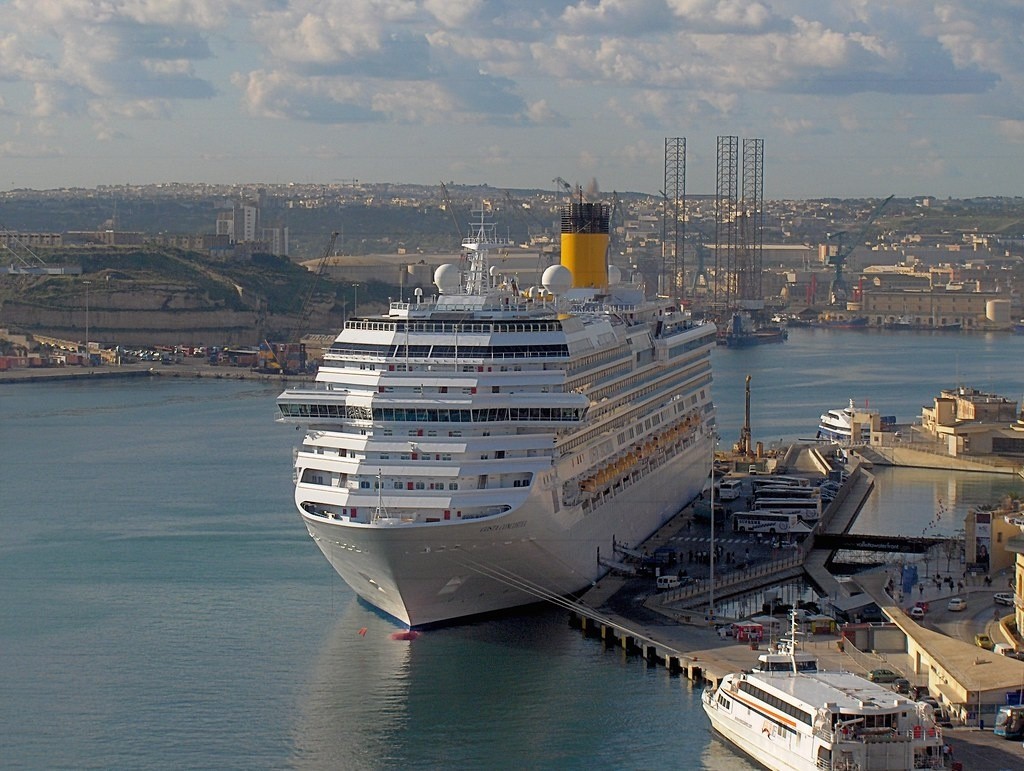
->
[276,182,721,634]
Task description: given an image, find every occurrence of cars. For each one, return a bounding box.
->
[974,633,993,651]
[910,606,925,620]
[947,599,969,612]
[933,708,952,725]
[868,669,902,684]
[917,696,938,708]
[816,471,847,503]
[892,679,910,695]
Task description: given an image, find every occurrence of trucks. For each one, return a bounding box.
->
[748,465,757,476]
[787,608,812,624]
[994,644,1018,660]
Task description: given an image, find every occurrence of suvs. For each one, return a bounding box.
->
[908,685,929,702]
[993,592,1014,606]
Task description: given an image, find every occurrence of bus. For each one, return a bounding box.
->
[993,705,1024,741]
[729,475,823,536]
[718,480,743,502]
[692,500,724,524]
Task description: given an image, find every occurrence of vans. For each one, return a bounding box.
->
[915,600,929,613]
[656,575,681,591]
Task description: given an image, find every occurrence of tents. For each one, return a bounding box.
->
[732,621,763,642]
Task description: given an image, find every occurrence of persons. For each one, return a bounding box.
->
[671,532,782,580]
[716,626,727,640]
[843,719,954,761]
[930,569,994,594]
[919,583,923,594]
[976,544,989,563]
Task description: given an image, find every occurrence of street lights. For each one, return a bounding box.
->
[81,281,92,360]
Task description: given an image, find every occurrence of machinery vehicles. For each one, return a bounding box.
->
[252,229,339,375]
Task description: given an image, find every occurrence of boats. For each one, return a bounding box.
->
[816,398,875,443]
[699,603,951,771]
[715,307,868,352]
